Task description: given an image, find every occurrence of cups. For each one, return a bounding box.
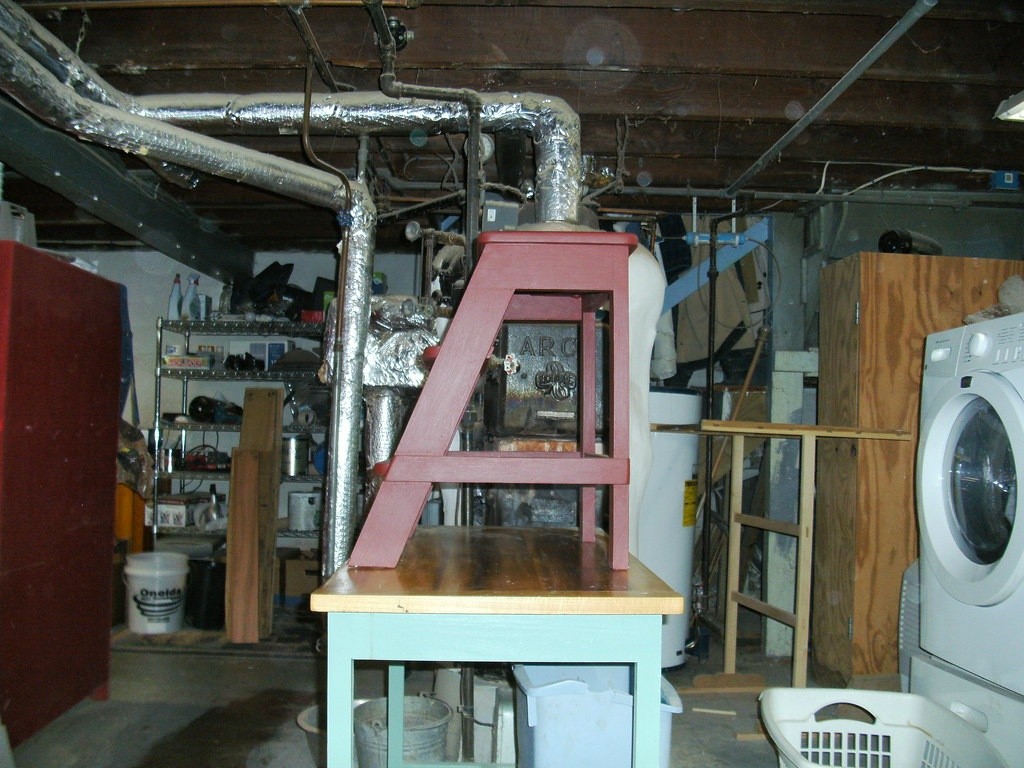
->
[166,344,187,355]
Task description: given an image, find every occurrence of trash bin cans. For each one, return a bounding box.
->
[513,664,683,768]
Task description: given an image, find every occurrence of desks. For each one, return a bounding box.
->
[309,524,684,767]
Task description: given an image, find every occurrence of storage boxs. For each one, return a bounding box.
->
[512,661,683,767]
[758,685,1010,767]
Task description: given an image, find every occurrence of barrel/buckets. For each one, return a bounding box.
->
[353,690,454,768]
[121,550,190,636]
[184,557,224,631]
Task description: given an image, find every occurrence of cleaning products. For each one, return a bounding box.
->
[164,271,203,321]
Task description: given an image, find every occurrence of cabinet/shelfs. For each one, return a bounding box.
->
[152,315,332,560]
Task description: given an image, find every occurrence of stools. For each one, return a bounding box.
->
[345,230,638,569]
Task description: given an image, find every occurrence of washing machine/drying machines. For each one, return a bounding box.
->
[913,311,1023,692]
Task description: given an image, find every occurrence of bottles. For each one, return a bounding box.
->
[168,274,183,321]
[205,483,224,523]
[196,344,225,369]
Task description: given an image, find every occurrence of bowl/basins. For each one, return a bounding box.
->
[296,698,373,768]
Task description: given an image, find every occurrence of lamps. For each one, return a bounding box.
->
[992,90,1023,122]
